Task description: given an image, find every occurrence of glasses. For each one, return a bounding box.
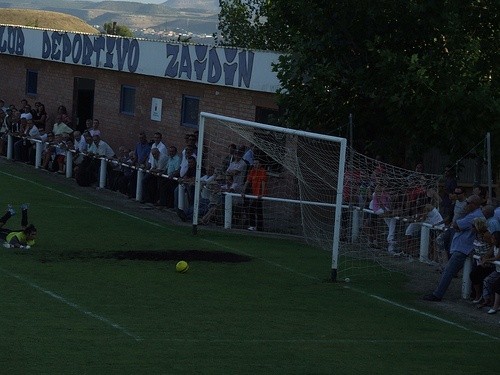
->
[455,192,463,195]
[466,200,473,205]
[47,135,52,137]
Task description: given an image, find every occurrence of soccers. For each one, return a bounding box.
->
[176,261,189,274]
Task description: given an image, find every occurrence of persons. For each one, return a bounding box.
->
[0,201,39,249]
[242,157,266,232]
[0,96,500,315]
[423,195,484,302]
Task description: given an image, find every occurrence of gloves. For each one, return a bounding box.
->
[20,245,30,250]
[3,242,14,249]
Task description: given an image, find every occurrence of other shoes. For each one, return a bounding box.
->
[430,294,442,302]
[22,204,28,213]
[248,226,256,231]
[472,293,500,313]
[8,204,16,216]
[178,209,187,221]
